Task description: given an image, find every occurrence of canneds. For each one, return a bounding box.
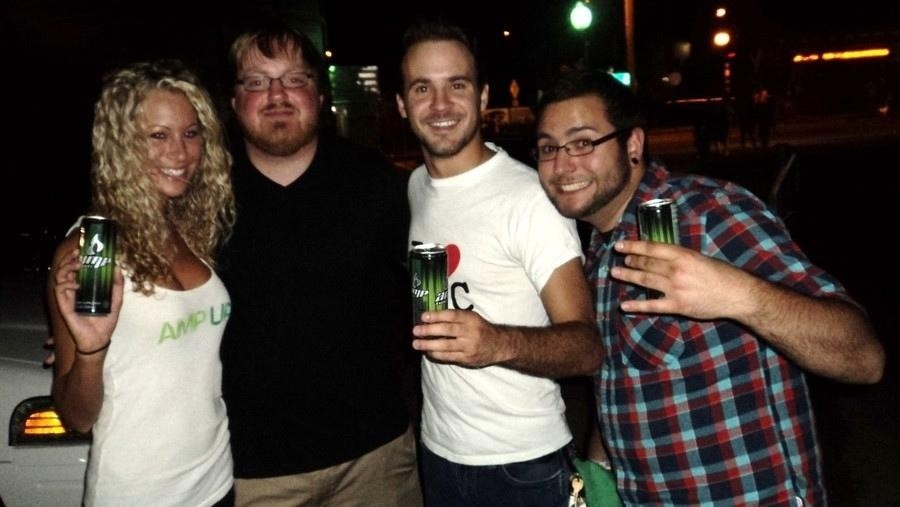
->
[74,216,117,313]
[639,197,679,244]
[409,246,448,325]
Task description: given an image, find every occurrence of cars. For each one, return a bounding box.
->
[0,305,91,507]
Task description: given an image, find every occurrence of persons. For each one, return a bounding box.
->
[45,17,889,506]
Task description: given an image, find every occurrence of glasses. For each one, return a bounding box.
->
[235,70,315,93]
[531,128,620,162]
